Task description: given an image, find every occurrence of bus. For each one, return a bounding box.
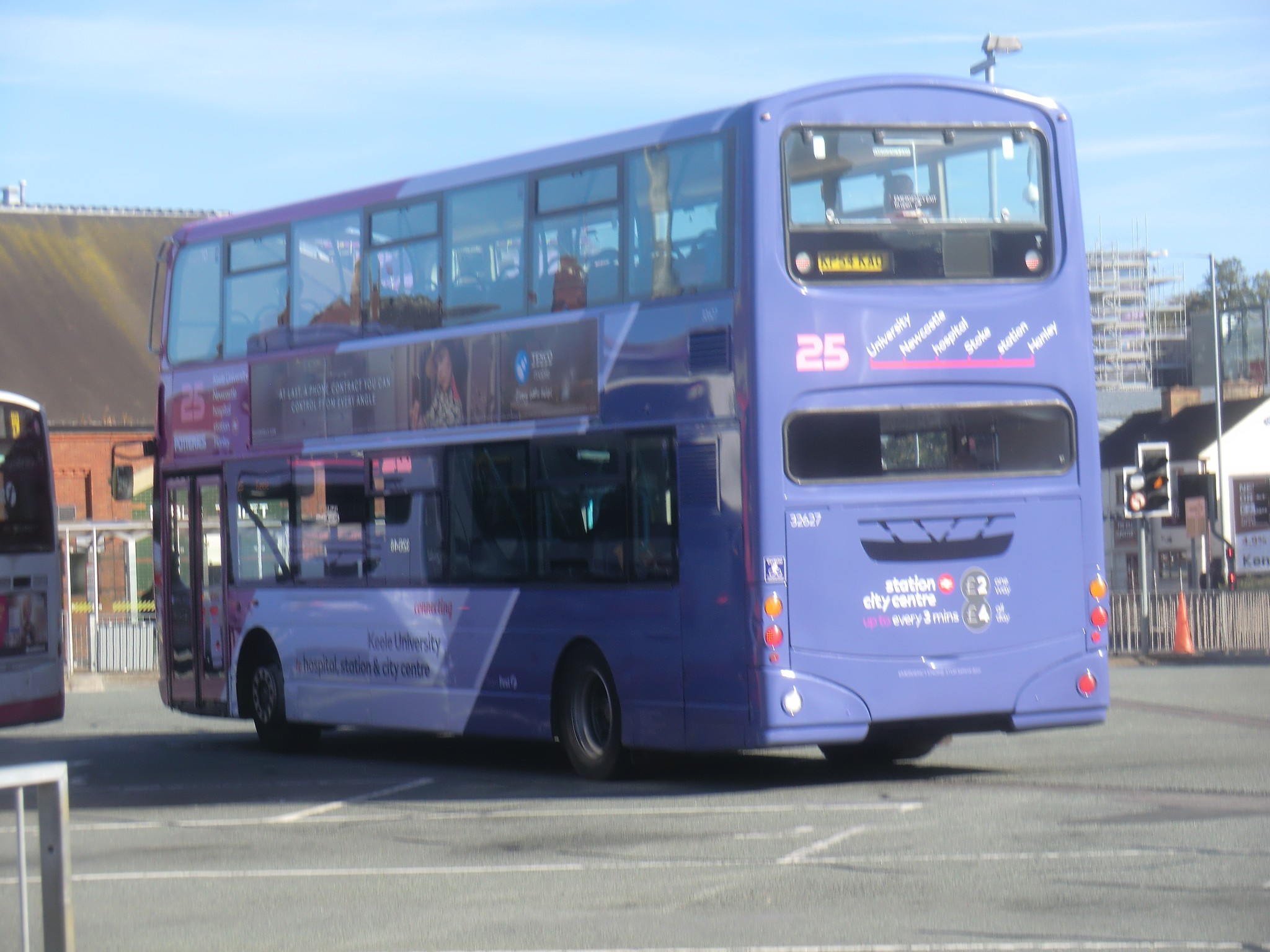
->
[107,74,1116,777]
[0,388,66,727]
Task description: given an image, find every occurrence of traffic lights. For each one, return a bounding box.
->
[1139,442,1172,519]
[1123,466,1147,518]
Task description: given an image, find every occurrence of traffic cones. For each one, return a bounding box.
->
[1169,590,1198,659]
